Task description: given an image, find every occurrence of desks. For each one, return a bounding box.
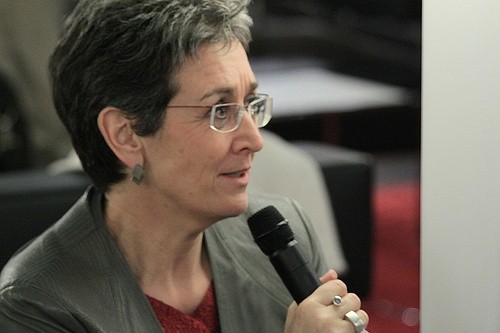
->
[253,64,412,117]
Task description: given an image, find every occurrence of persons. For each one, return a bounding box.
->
[0,0,375,333]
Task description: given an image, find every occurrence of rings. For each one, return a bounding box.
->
[346,311,363,333]
[333,295,343,306]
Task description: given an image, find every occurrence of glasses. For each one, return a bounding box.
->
[116,93,275,133]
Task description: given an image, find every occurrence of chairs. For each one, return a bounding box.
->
[244,130,350,278]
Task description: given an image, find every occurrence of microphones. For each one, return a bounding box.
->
[247,205,319,306]
